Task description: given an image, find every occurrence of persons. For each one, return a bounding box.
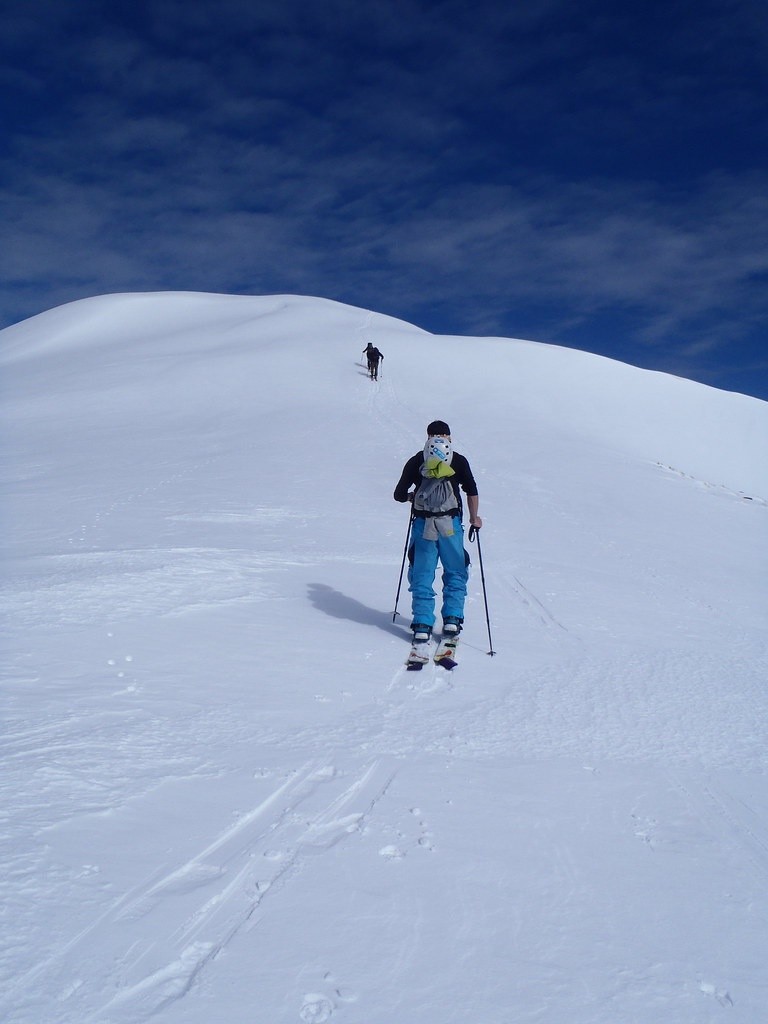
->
[363,343,373,370]
[394,420,483,640]
[369,347,384,378]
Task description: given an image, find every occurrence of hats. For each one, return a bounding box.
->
[427,420,451,435]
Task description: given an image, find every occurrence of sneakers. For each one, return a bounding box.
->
[444,616,464,634]
[410,623,433,639]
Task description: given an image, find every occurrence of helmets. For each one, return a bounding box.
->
[424,437,453,466]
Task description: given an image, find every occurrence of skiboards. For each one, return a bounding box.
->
[403,613,462,665]
[368,364,379,382]
